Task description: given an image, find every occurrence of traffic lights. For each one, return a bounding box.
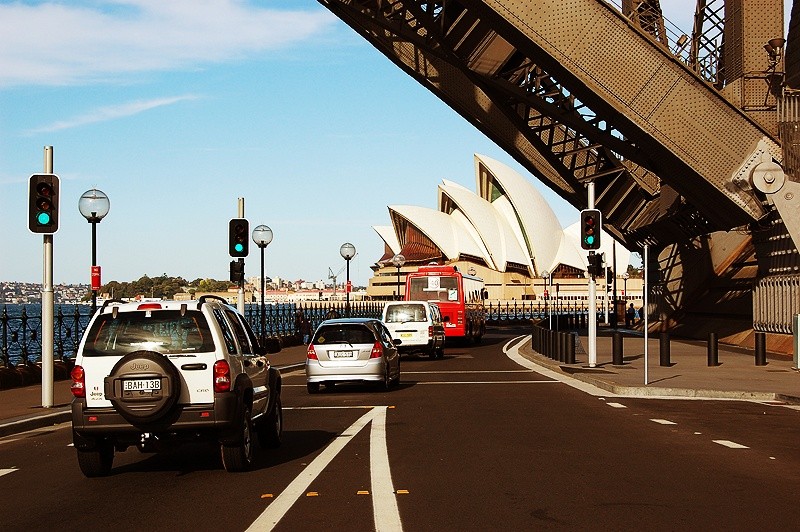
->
[580,209,602,250]
[586,253,603,277]
[607,285,613,292]
[27,173,63,234]
[606,267,614,284]
[229,261,244,283]
[228,219,250,259]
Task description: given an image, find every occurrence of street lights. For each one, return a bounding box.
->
[622,272,630,303]
[77,188,111,317]
[339,243,357,317]
[392,254,406,302]
[540,270,550,320]
[251,225,274,349]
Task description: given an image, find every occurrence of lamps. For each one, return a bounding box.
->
[763,37,786,73]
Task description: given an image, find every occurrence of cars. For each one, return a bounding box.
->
[304,318,403,394]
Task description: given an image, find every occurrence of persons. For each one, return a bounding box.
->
[628,303,636,327]
[295,307,340,347]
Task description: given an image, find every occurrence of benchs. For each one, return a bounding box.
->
[121,329,203,347]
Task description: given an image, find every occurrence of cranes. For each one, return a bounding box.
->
[328,252,359,297]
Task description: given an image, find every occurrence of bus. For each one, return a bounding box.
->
[405,263,491,342]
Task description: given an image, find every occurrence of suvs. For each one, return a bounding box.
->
[381,300,450,359]
[70,295,284,473]
